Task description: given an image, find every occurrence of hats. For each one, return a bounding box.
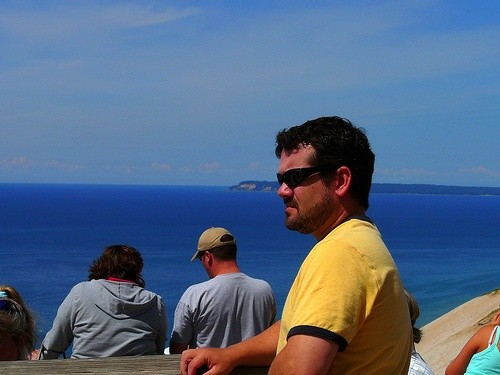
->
[190,227,236,262]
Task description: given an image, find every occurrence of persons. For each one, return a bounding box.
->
[168,228,277,353]
[0,286,37,360]
[180,115,414,375]
[444,309,500,375]
[403,289,437,375]
[27,244,168,359]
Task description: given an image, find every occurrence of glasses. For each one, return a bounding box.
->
[277,162,362,189]
[0,300,21,316]
